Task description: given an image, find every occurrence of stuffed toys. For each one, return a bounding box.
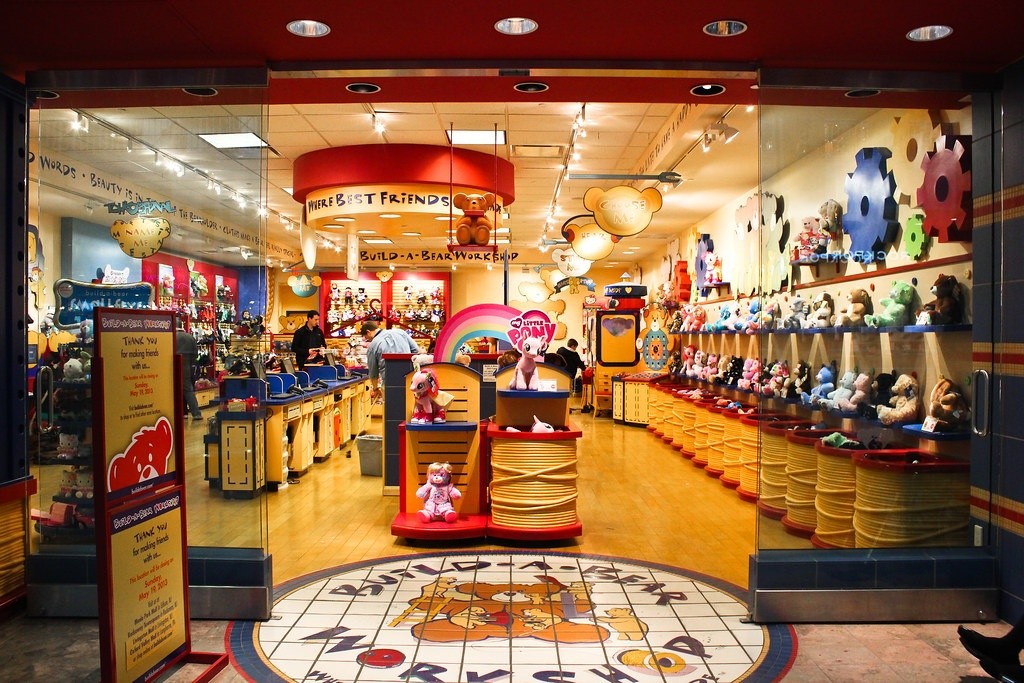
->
[389,282,443,341]
[703,251,722,283]
[410,363,454,425]
[506,414,563,432]
[415,461,461,523]
[57,318,94,531]
[410,354,472,371]
[656,284,667,299]
[452,192,496,247]
[327,282,383,337]
[667,273,972,448]
[509,329,548,390]
[161,272,266,390]
[795,198,843,255]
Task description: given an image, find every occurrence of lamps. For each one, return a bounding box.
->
[701,119,740,152]
[669,176,683,189]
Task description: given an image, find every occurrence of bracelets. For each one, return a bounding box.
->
[373,387,379,392]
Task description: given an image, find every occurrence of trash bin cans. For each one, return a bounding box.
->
[357,436,383,477]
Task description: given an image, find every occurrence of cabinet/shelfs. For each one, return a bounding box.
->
[391,362,487,540]
[486,362,582,541]
[39,343,95,554]
[669,325,973,440]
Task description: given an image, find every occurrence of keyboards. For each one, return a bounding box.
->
[338,377,353,380]
[303,387,321,392]
[270,393,296,399]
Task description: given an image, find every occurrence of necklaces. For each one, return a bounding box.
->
[373,329,383,338]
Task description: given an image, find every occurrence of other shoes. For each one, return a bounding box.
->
[183,414,188,418]
[194,415,203,419]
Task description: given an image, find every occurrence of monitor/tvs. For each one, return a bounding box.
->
[249,362,267,380]
[280,359,295,374]
[323,353,336,367]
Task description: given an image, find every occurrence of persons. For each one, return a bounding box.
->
[556,339,586,393]
[291,309,330,372]
[176,321,204,421]
[958,612,1024,683]
[360,322,419,400]
